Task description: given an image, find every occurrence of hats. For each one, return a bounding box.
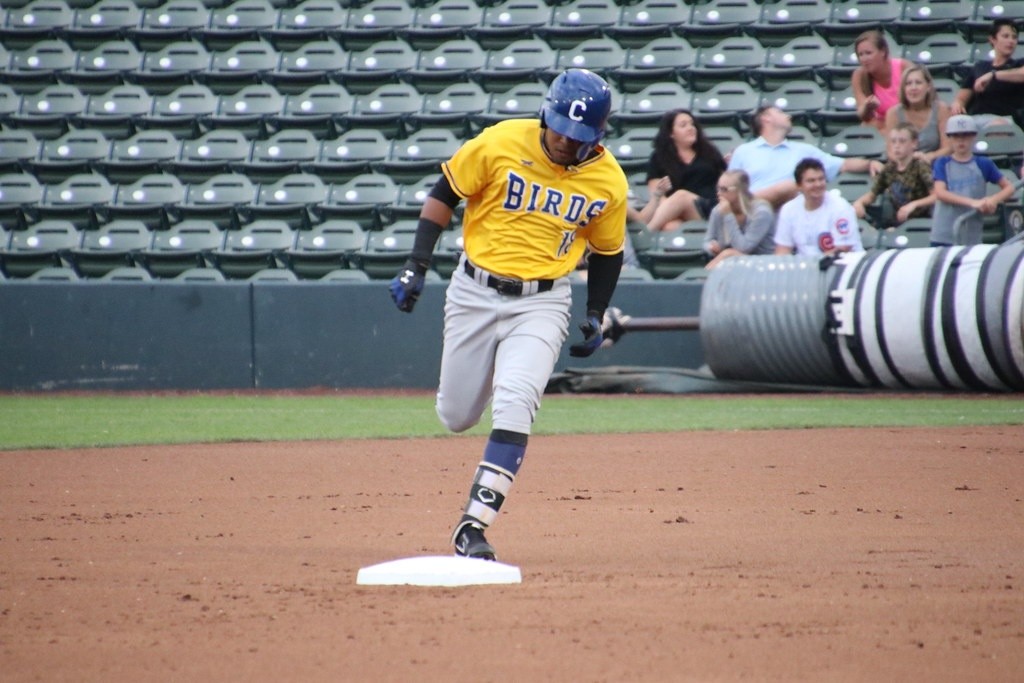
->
[945,114,977,136]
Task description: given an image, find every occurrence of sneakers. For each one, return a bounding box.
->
[455,524,497,562]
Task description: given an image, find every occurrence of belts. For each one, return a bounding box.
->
[465,260,553,295]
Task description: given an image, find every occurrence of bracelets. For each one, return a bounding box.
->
[654,191,663,197]
[991,70,997,79]
[733,208,743,215]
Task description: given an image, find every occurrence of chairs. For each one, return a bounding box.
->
[0,0,1024,283]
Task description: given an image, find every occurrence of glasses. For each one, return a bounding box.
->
[717,185,737,193]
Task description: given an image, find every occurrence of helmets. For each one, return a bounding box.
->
[539,68,612,159]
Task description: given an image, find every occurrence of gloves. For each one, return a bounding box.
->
[568,310,604,357]
[388,259,426,312]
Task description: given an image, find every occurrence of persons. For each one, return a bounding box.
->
[928,114,1016,249]
[851,31,918,136]
[694,170,779,281]
[726,103,885,214]
[951,16,1024,132]
[575,176,672,270]
[389,67,629,563]
[885,64,955,167]
[773,158,869,257]
[853,121,937,232]
[637,108,729,236]
